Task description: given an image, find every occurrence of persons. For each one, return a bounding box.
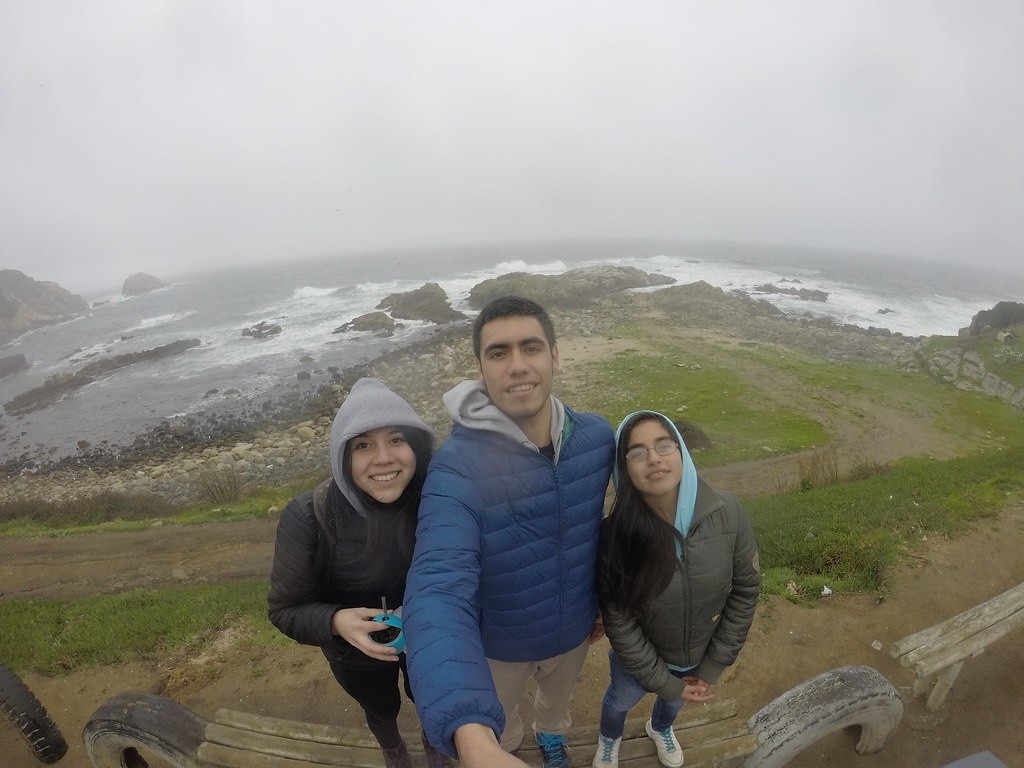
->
[403,297,617,768]
[268,379,456,768]
[595,409,760,768]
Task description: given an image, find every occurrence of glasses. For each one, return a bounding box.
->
[625,442,678,462]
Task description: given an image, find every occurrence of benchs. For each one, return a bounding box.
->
[891,581,1024,711]
[82,665,904,768]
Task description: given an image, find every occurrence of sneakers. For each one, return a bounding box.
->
[592,733,622,768]
[645,719,684,768]
[534,730,572,768]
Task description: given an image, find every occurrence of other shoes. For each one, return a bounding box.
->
[382,736,414,768]
[420,729,453,768]
